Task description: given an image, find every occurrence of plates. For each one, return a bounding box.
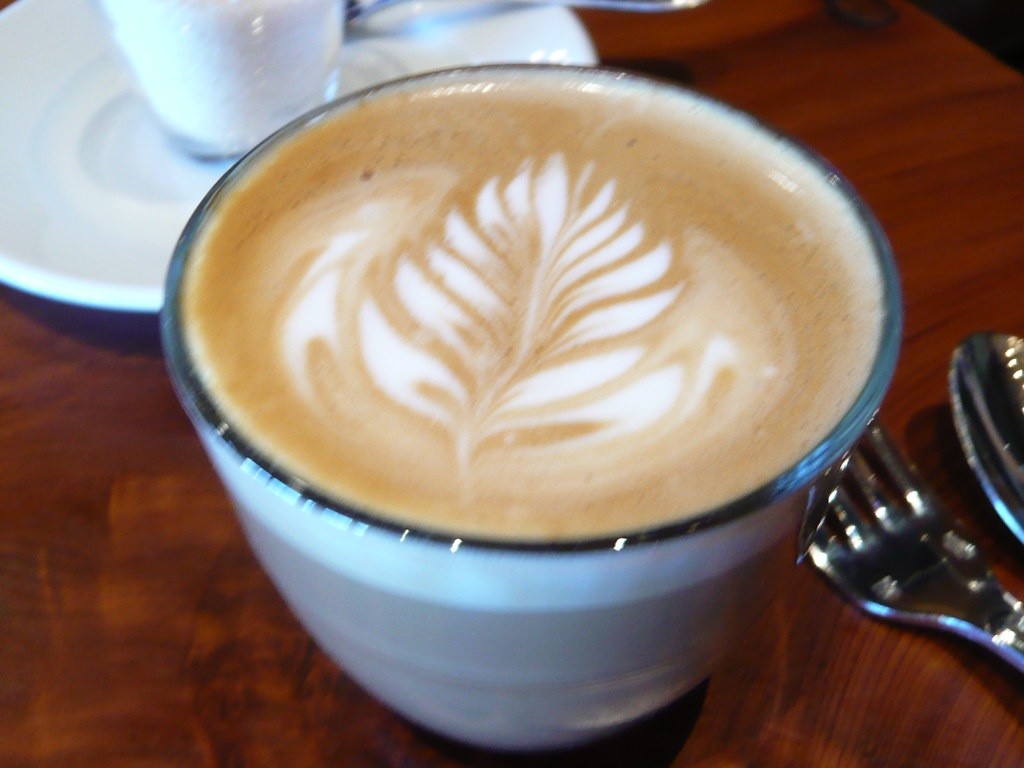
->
[0,0,599,314]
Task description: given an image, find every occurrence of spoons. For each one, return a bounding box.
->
[943,332,1024,547]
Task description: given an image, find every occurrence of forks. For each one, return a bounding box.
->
[796,418,1024,674]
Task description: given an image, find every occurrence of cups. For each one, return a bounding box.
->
[161,61,903,753]
[100,0,347,165]
